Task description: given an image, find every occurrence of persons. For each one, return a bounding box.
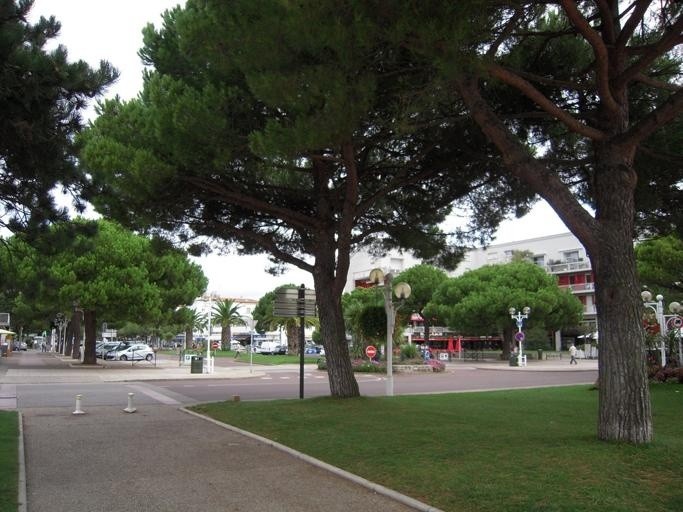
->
[192,342,201,349]
[417,342,429,353]
[234,344,241,359]
[569,343,577,364]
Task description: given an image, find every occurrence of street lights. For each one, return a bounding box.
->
[642,289,681,371]
[370,266,411,397]
[507,305,529,364]
[54,313,64,355]
[202,289,219,374]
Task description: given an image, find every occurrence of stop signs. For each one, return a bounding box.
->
[365,346,378,360]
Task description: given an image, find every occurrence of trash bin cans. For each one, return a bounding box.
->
[2,345,7,357]
[510,357,516,366]
[538,349,543,360]
[191,356,203,373]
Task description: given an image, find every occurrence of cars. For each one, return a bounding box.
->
[54,338,324,363]
[13,340,27,351]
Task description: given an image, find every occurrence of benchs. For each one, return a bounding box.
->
[546,352,562,360]
